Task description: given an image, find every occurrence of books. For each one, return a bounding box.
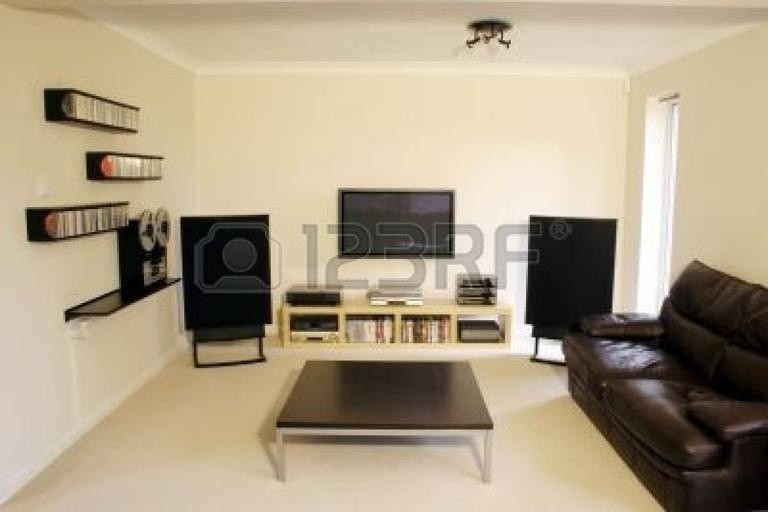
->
[43,92,163,239]
[346,315,451,344]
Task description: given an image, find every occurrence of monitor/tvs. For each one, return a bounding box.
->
[338,188,456,260]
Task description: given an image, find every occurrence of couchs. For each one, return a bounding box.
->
[563,257,768,512]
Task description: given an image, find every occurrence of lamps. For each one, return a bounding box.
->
[466,20,513,49]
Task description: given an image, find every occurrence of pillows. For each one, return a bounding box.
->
[658,261,762,386]
[706,288,768,400]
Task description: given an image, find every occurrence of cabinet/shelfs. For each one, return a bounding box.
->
[26,87,181,324]
[281,296,511,351]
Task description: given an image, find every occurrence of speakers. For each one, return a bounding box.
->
[525,216,618,329]
[179,213,272,331]
